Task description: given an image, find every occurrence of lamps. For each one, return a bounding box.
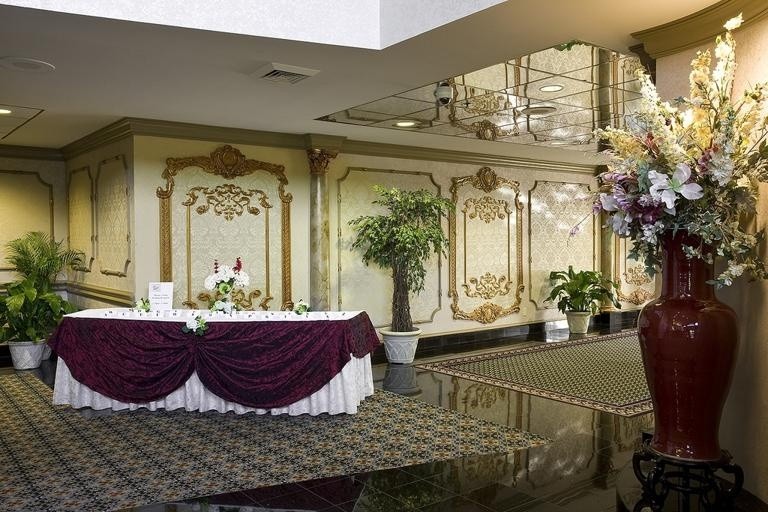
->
[394,122,415,128]
[522,107,556,116]
[538,86,562,93]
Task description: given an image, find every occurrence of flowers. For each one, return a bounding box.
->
[577,10,768,290]
[181,264,250,338]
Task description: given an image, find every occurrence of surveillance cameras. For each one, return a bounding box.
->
[435,82,454,106]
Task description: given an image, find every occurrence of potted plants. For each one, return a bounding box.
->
[549,264,623,334]
[346,183,458,365]
[0,230,83,371]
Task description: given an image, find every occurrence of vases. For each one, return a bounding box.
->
[634,227,738,462]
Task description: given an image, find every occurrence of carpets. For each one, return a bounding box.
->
[418,327,654,417]
[0,370,557,512]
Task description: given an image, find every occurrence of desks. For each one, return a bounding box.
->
[632,436,745,512]
[56,308,368,414]
